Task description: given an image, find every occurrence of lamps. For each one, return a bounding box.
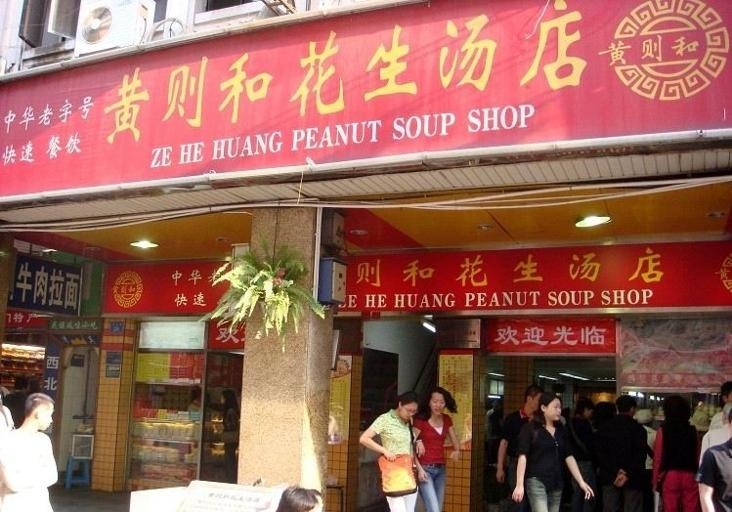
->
[420,318,436,333]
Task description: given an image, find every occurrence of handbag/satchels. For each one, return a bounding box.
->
[377,454,417,497]
[221,431,238,443]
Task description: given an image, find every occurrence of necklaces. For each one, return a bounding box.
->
[428,417,444,427]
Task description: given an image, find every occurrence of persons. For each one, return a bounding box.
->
[0,392,59,512]
[485,380,732,512]
[219,387,237,484]
[187,388,209,411]
[275,486,319,511]
[0,375,42,437]
[309,488,324,511]
[358,390,427,512]
[413,386,460,512]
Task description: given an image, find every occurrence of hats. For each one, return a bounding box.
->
[633,409,653,424]
[689,412,709,432]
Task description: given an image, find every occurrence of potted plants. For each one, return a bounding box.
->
[197,198,329,354]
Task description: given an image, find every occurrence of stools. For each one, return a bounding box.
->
[64,453,90,489]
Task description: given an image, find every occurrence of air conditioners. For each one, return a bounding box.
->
[74,0,156,57]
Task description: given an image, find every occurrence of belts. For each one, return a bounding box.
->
[425,464,442,468]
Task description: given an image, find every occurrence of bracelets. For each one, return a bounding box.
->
[414,439,423,443]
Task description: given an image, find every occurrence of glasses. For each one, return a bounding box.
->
[402,406,416,413]
